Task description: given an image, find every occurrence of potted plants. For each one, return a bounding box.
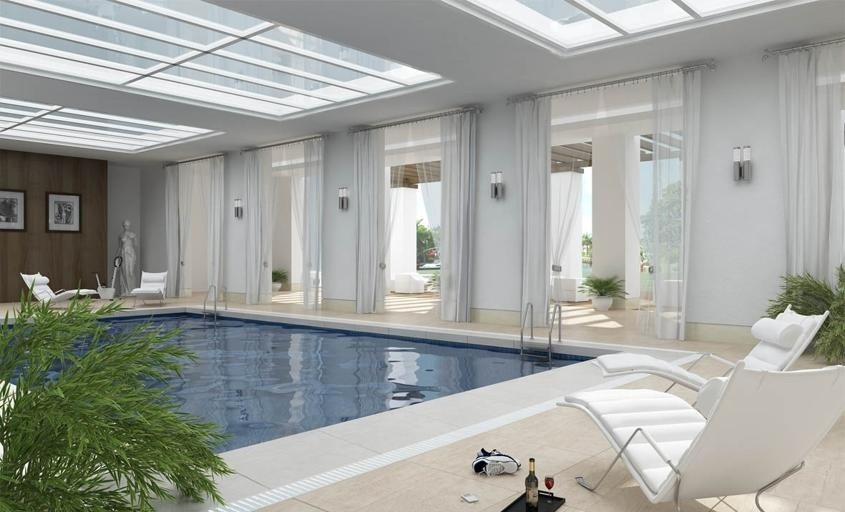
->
[272,268,288,292]
[577,271,629,311]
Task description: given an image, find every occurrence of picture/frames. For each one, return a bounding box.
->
[0,189,28,233]
[45,190,83,234]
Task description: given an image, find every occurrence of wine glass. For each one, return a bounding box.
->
[544,476,555,504]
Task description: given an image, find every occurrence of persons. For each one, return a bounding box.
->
[114,219,139,294]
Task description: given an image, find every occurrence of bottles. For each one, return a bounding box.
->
[525,458,539,511]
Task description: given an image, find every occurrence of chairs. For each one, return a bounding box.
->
[590,302,829,407]
[18,270,100,307]
[659,280,685,341]
[131,268,168,307]
[555,356,845,512]
[554,276,590,302]
[394,272,427,293]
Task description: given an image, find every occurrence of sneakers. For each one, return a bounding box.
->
[471,448,522,475]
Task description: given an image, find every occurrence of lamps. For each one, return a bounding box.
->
[234,198,243,218]
[338,187,348,209]
[731,145,753,183]
[490,170,504,201]
